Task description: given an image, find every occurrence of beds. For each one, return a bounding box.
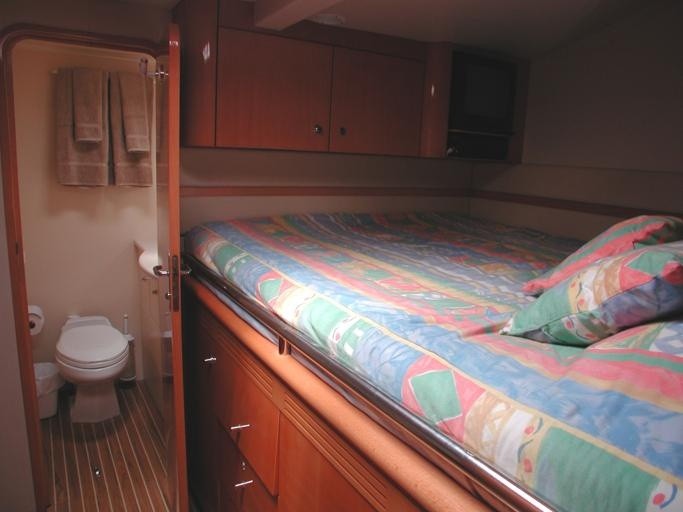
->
[181,211,681,511]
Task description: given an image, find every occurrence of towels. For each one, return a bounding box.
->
[52,68,154,191]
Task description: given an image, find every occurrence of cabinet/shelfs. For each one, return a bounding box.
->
[136,252,171,426]
[419,43,533,164]
[169,2,448,159]
[183,286,421,509]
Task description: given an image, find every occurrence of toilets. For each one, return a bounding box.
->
[55,316,130,424]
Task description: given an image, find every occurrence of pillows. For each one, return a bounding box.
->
[498,214,682,343]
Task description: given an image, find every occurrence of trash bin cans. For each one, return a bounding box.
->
[34,362,59,420]
